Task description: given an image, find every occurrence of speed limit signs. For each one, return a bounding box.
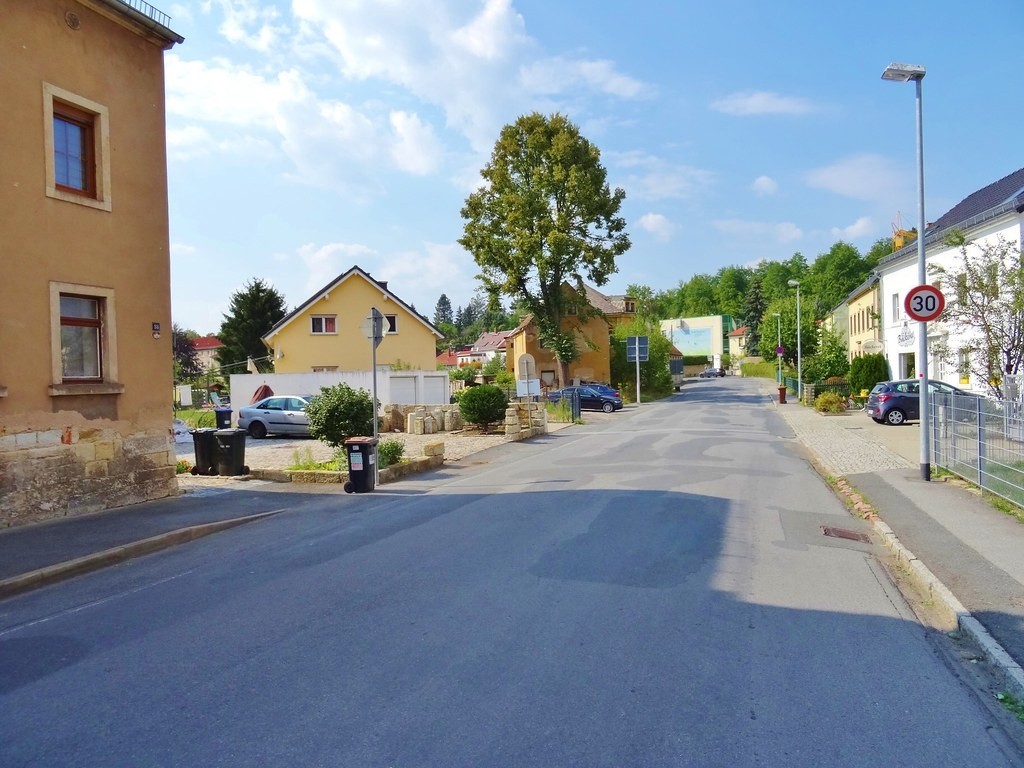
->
[903,285,945,323]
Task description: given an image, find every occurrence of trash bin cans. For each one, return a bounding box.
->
[213,427,250,476]
[214,408,233,429]
[778,386,787,404]
[342,436,379,493]
[188,428,219,476]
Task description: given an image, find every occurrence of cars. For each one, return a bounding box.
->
[237,393,322,439]
[866,378,987,426]
[547,383,624,414]
[699,368,726,378]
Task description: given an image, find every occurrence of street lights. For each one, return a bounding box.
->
[786,278,802,401]
[772,312,783,385]
[881,61,938,482]
